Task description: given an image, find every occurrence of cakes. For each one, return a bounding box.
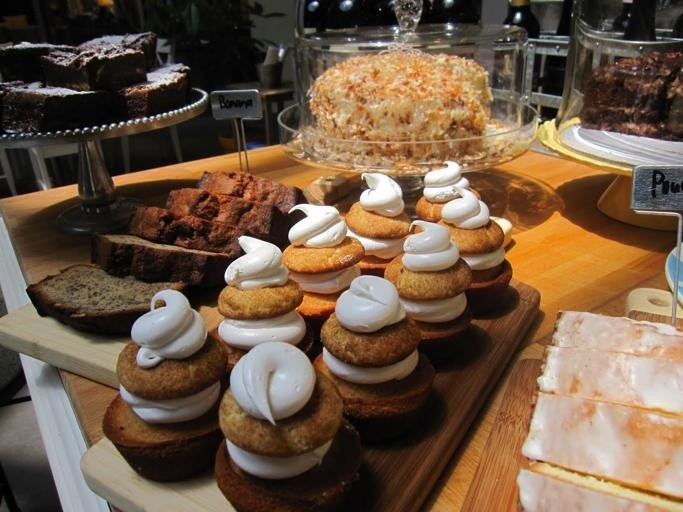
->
[342,171,412,279]
[218,234,316,381]
[282,203,366,326]
[310,274,437,440]
[105,289,231,483]
[384,219,472,367]
[437,185,510,313]
[214,341,362,511]
[579,50,683,141]
[415,162,482,225]
[307,50,493,163]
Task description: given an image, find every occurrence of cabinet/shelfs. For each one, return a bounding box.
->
[1,106,683,512]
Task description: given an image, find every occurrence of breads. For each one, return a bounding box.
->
[24,171,309,340]
[516,310,683,512]
[0,30,191,128]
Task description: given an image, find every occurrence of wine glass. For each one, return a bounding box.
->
[275,89,543,210]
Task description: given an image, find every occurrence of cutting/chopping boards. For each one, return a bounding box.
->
[464,310,682,512]
[3,275,540,512]
